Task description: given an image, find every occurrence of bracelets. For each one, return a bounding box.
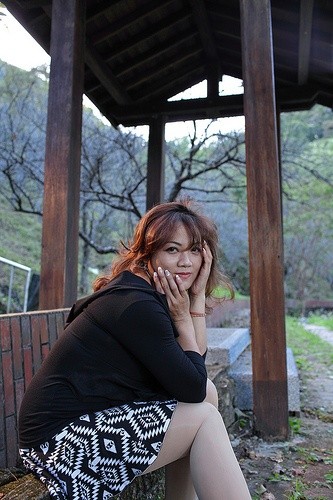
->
[189,311,207,318]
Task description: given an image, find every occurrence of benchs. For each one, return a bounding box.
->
[0,473,50,500]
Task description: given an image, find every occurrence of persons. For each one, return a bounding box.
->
[18,202,253,500]
